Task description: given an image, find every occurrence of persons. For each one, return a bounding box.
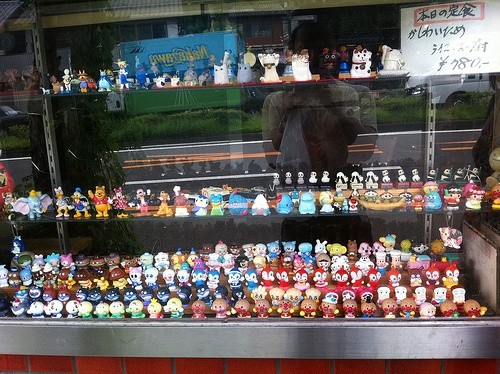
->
[338,46,350,73]
[261,20,379,246]
[471,72,500,186]
[117,61,129,89]
[183,55,198,80]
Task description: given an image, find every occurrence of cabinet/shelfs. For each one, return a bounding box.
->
[0,0,500,361]
